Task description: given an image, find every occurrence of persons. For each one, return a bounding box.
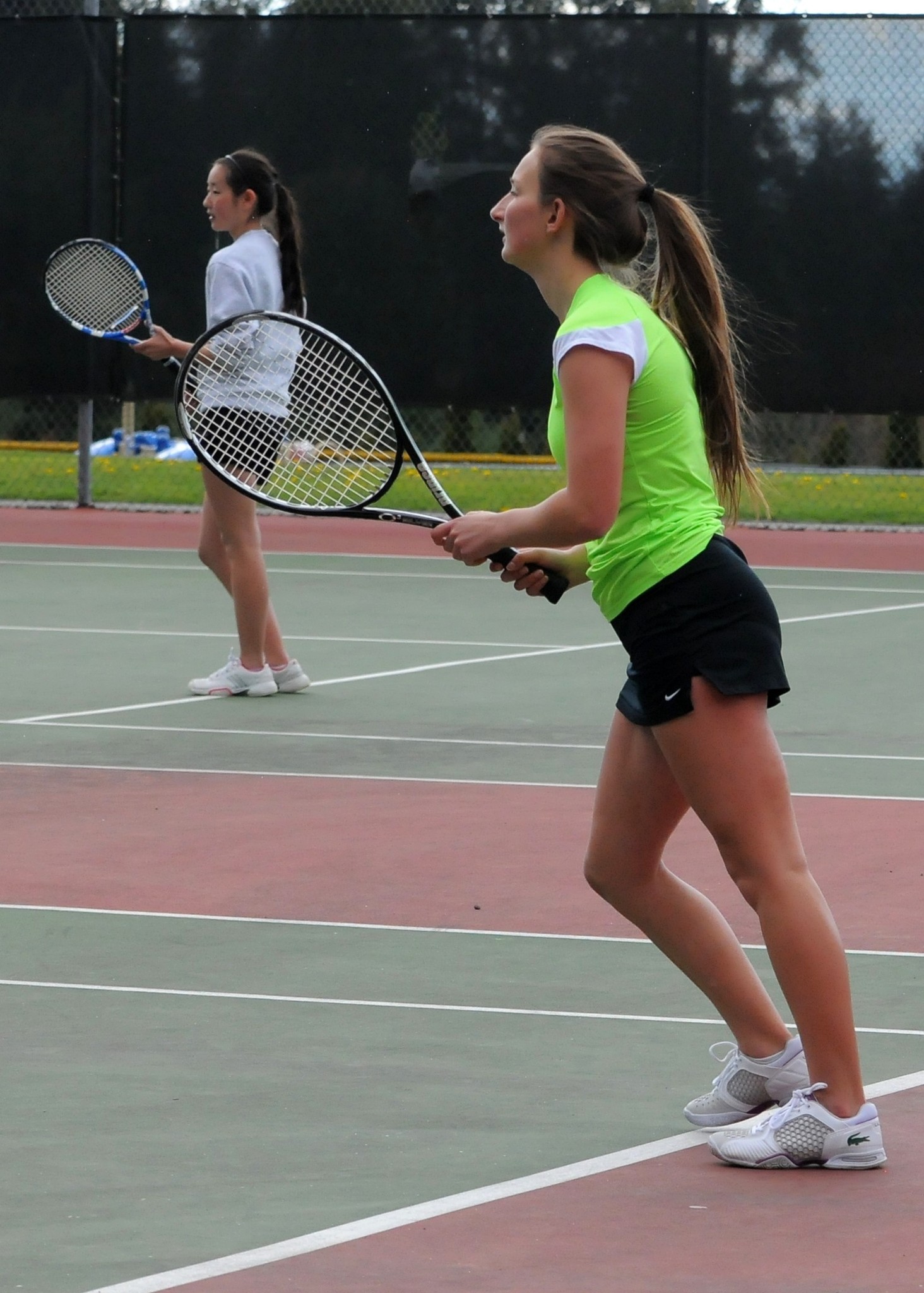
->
[128,150,311,697]
[431,125,887,1171]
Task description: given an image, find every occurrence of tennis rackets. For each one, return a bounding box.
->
[41,238,198,398]
[172,310,572,605]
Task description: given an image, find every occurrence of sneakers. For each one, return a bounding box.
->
[707,1080,885,1170]
[188,647,278,697]
[683,1033,811,1127]
[270,658,310,693]
